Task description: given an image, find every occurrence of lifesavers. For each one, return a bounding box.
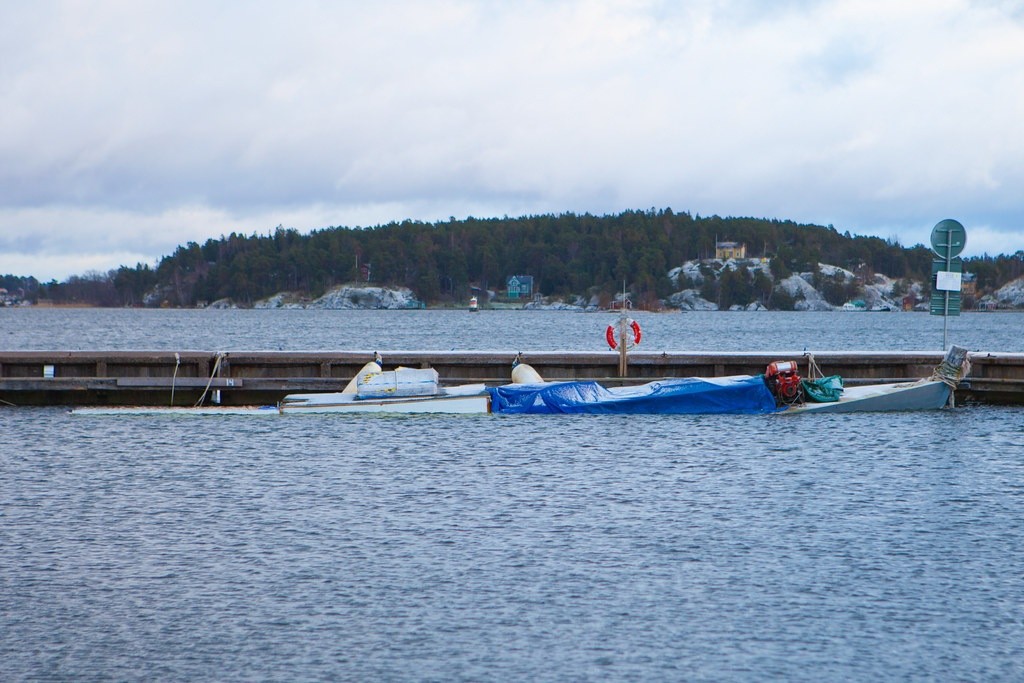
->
[782,383,797,397]
[604,317,643,352]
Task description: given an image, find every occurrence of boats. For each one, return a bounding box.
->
[278,346,967,415]
